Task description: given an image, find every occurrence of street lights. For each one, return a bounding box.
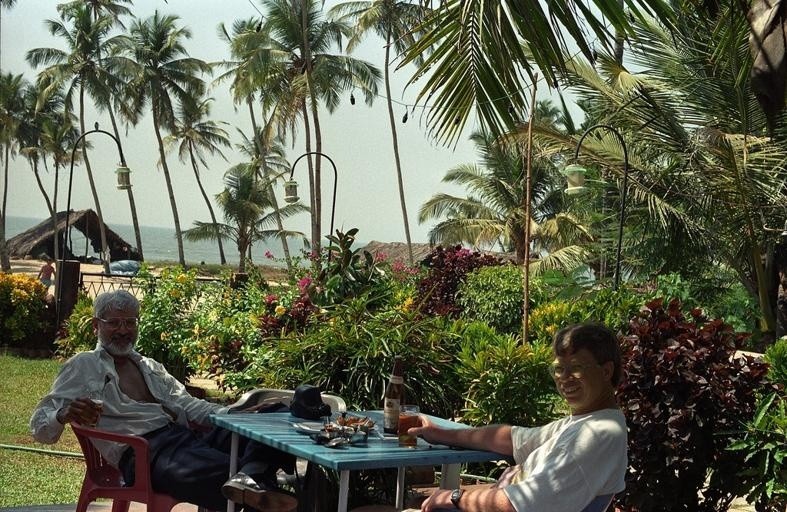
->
[59,124,132,323]
[285,151,338,266]
[565,125,628,290]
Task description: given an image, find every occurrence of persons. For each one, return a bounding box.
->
[29,291,298,512]
[397,322,628,512]
[36,259,56,289]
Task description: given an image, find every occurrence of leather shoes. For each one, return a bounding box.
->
[221,472,298,512]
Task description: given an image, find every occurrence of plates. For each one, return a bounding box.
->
[293,422,332,434]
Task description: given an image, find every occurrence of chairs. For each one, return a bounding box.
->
[217,388,347,511]
[65,417,187,512]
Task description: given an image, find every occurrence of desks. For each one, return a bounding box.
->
[209,406,508,511]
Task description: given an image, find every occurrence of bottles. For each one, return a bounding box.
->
[384,354,404,435]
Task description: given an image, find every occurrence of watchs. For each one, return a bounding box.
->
[451,486,465,510]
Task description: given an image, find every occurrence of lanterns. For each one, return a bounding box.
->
[114,166,133,190]
[282,180,300,203]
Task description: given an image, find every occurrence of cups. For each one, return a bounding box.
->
[86,387,103,428]
[398,404,420,449]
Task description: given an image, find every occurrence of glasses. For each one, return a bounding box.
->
[97,315,140,329]
[547,361,589,379]
